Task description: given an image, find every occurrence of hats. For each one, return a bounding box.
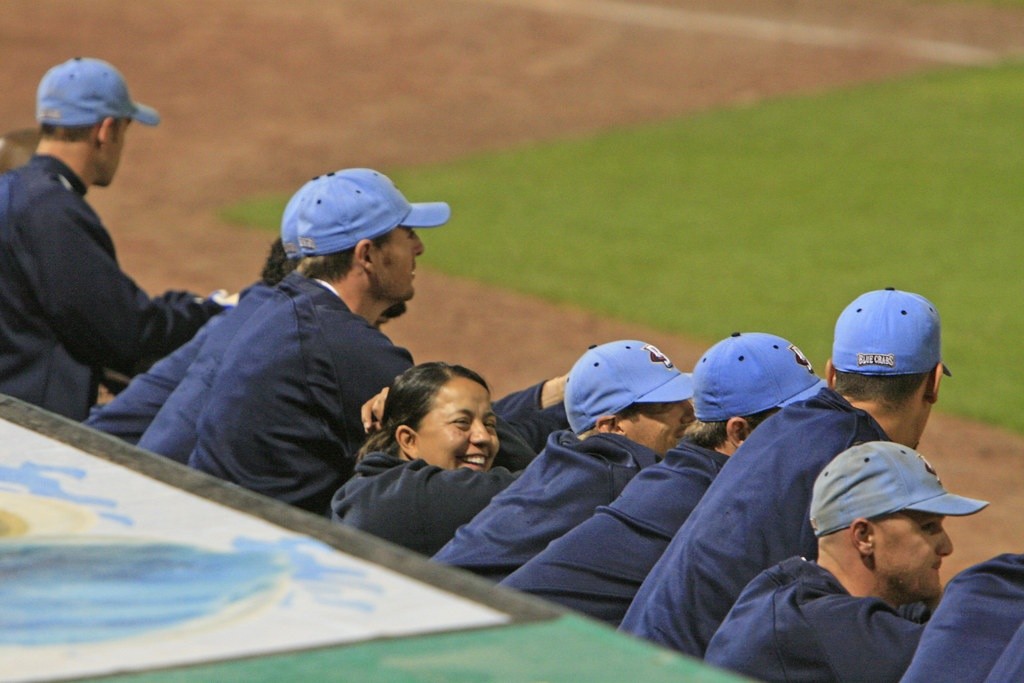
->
[693,332,829,421]
[564,340,695,437]
[810,441,990,537]
[280,174,326,259]
[36,56,160,126]
[297,168,451,256]
[832,287,952,377]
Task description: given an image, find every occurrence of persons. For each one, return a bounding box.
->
[2,58,1024,682]
